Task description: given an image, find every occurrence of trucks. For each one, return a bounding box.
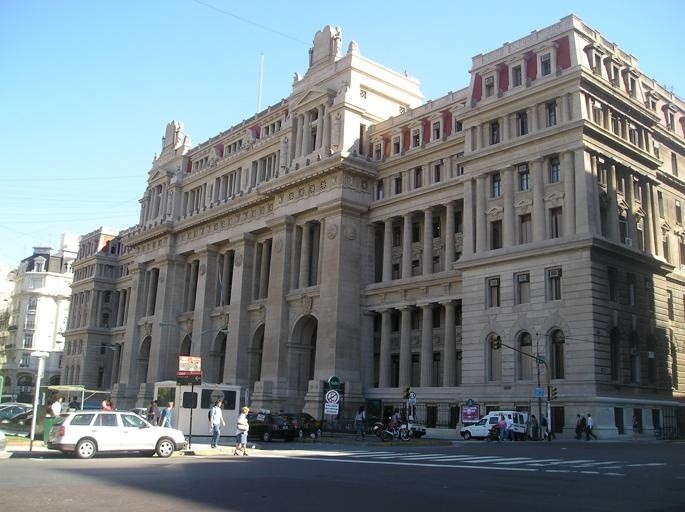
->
[460,410,529,441]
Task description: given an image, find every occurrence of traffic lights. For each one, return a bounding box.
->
[492,334,502,350]
[549,385,558,399]
[403,386,410,398]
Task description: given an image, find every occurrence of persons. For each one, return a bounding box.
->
[210,399,225,448]
[390,408,403,429]
[49,396,174,428]
[498,411,597,441]
[234,406,250,456]
[354,406,367,441]
[629,415,639,440]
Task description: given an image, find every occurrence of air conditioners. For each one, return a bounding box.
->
[625,238,633,247]
[645,281,651,289]
[637,223,643,230]
[630,347,637,355]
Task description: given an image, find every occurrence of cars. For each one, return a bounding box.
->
[129,407,148,420]
[277,412,322,438]
[0,400,105,437]
[0,431,7,450]
[46,411,187,458]
[247,412,300,441]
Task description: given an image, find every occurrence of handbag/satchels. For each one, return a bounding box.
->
[237,423,249,431]
[362,417,365,423]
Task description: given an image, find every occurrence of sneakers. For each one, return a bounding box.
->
[243,453,248,456]
[234,452,239,455]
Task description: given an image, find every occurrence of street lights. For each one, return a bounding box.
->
[28,350,49,448]
[158,321,230,354]
[56,340,120,364]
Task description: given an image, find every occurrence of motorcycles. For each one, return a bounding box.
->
[371,415,386,438]
[381,423,411,442]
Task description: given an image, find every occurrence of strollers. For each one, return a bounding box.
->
[487,424,501,442]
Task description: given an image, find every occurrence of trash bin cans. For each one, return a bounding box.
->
[43,416,56,446]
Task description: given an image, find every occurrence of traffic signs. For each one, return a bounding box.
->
[324,403,339,409]
[324,408,339,414]
[176,375,202,385]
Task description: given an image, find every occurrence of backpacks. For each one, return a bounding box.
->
[542,417,547,427]
